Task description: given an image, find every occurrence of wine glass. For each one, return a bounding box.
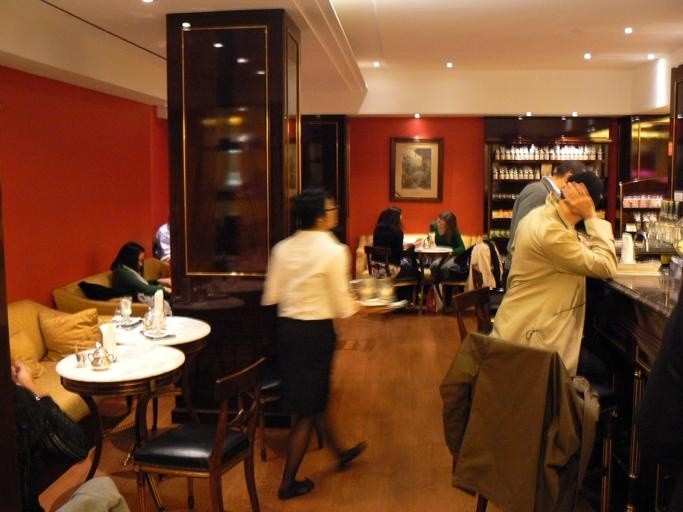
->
[118,296,132,323]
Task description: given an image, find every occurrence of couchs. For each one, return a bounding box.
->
[51,257,171,317]
[7,298,113,424]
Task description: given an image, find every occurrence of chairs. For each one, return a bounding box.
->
[362,244,419,312]
[441,242,490,312]
[132,351,267,511]
[437,331,622,511]
[451,286,490,344]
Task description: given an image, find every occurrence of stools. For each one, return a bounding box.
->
[234,370,324,464]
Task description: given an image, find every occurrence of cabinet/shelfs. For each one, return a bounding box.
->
[484,135,613,255]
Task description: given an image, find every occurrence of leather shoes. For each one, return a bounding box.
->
[340,442,366,467]
[278,479,315,499]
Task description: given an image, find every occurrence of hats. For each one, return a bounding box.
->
[571,170,605,204]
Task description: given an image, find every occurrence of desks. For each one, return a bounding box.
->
[165,292,246,416]
[192,274,270,351]
[97,314,212,466]
[403,246,453,308]
[53,344,187,511]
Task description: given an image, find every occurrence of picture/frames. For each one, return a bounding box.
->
[387,135,444,205]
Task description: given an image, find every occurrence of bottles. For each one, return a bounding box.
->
[621,196,683,294]
[495,144,603,162]
[492,167,541,180]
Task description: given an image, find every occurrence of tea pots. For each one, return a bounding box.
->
[86,345,115,370]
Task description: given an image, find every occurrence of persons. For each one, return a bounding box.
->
[260,187,371,501]
[111,241,167,300]
[417,207,466,315]
[635,280,683,480]
[10,356,98,511]
[500,160,587,294]
[487,168,620,393]
[372,203,421,314]
[152,221,170,263]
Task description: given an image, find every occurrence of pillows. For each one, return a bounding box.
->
[75,279,123,301]
[36,306,102,363]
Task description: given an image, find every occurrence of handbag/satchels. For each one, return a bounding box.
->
[448,264,469,281]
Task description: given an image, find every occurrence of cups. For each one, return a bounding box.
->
[428,232,436,248]
[358,274,394,303]
